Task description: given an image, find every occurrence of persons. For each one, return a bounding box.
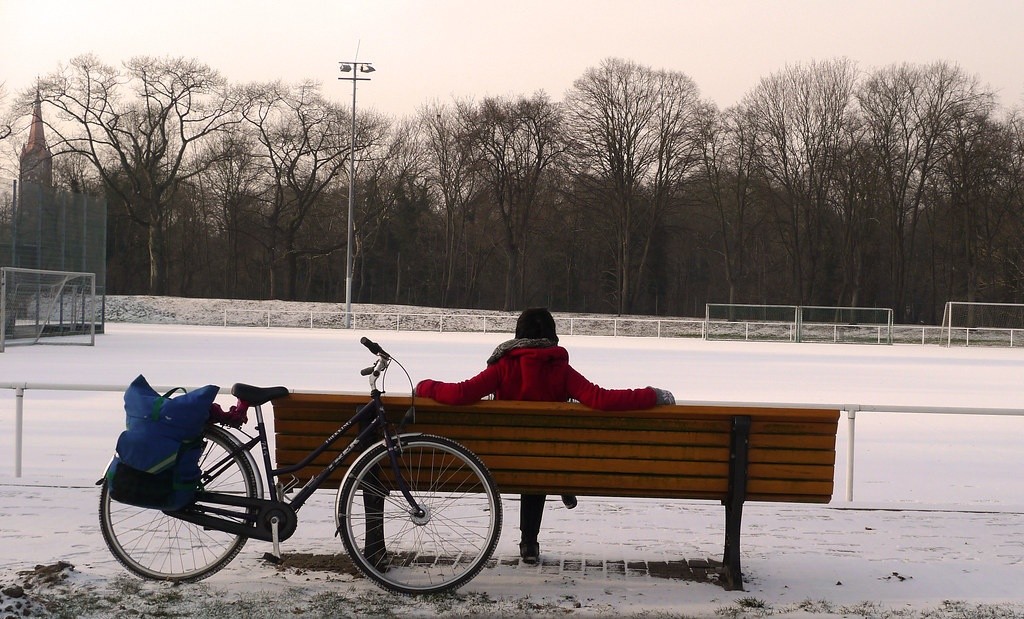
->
[414,308,677,563]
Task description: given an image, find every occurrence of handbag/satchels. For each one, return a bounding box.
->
[107,375,220,510]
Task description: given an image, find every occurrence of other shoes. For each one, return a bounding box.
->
[560,492,577,510]
[520,541,540,561]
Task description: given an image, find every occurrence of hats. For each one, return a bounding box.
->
[515,307,557,341]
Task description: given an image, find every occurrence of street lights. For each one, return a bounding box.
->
[338,60,376,325]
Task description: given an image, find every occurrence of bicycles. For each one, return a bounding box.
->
[95,336,504,597]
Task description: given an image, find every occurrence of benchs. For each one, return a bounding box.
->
[265,392,838,593]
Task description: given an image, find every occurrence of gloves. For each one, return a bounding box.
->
[647,385,676,405]
